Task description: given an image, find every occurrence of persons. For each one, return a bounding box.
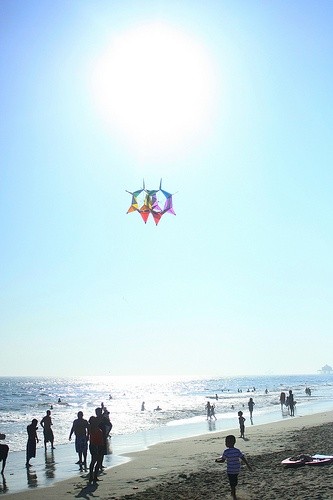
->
[209,405,217,421]
[86,402,113,486]
[305,387,312,396]
[247,398,255,420]
[109,394,112,400]
[40,410,56,452]
[68,411,89,468]
[50,405,53,409]
[215,387,269,401]
[58,398,61,402]
[215,435,254,500]
[280,389,297,417]
[156,406,161,410]
[205,401,213,420]
[27,419,39,468]
[237,411,246,438]
[141,402,146,411]
[0,444,9,475]
